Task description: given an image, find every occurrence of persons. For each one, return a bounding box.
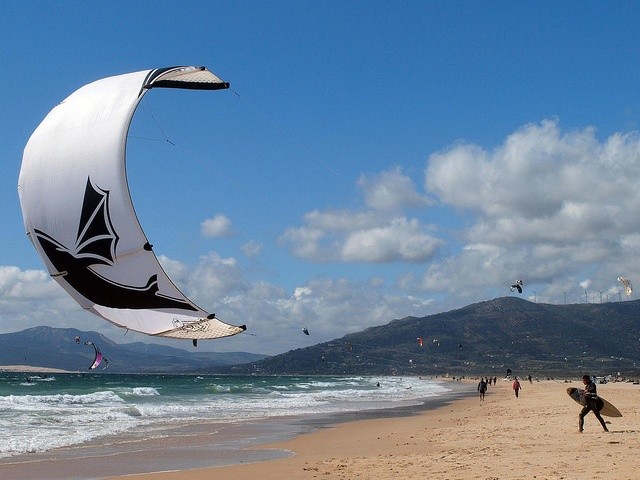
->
[485,375,496,386]
[577,374,609,433]
[512,377,522,399]
[528,374,533,383]
[376,382,381,386]
[477,377,487,402]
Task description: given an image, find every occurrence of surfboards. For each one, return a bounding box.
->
[566,387,623,417]
